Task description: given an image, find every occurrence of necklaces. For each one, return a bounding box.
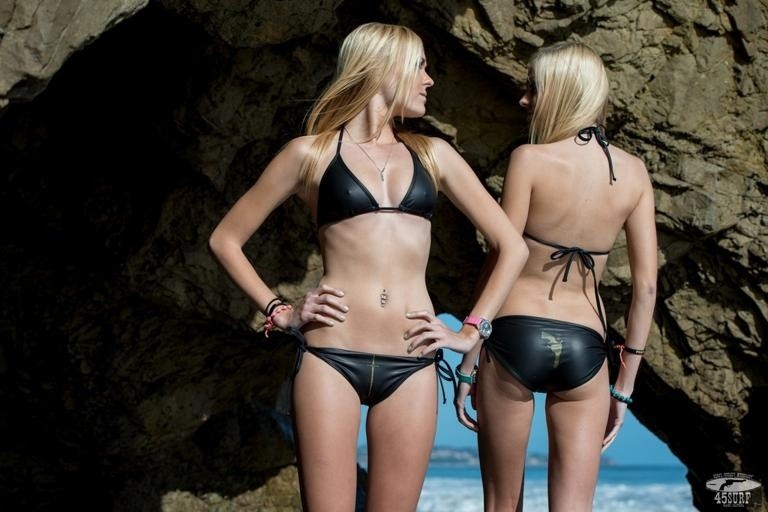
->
[344,127,394,182]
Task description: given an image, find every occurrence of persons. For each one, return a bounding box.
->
[453,40,659,512]
[208,21,530,512]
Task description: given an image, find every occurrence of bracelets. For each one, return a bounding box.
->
[610,385,633,405]
[622,345,646,355]
[264,297,293,324]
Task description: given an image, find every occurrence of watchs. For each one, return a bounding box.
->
[455,364,479,385]
[463,316,493,340]
